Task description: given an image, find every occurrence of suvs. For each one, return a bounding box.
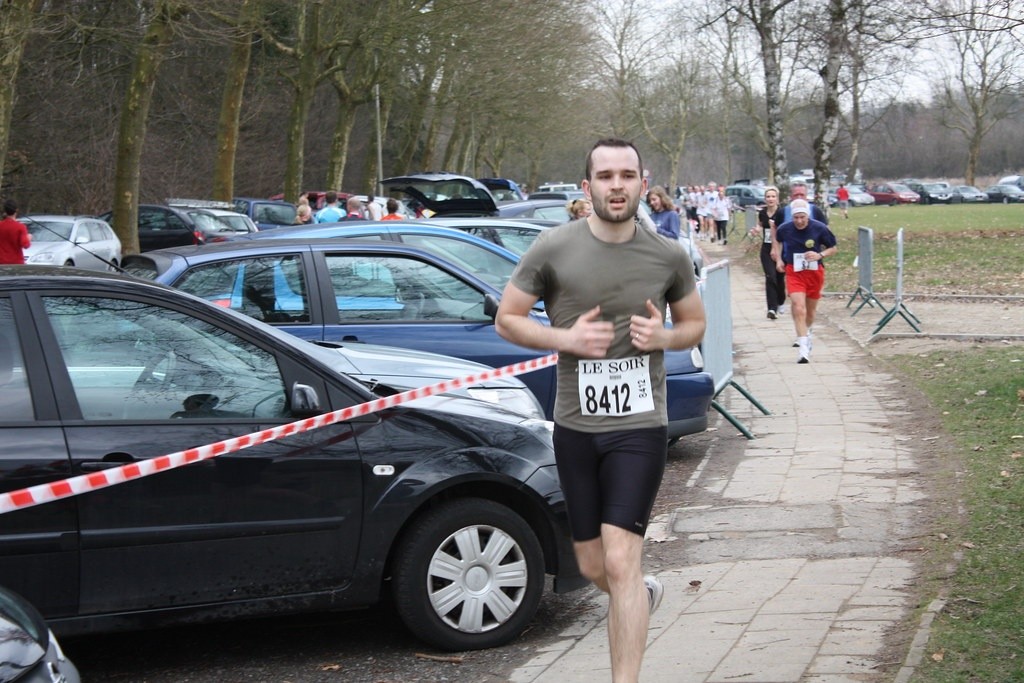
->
[15,214,123,274]
[98,191,436,253]
[908,181,953,204]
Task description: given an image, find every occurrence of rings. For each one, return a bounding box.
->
[635,332,640,339]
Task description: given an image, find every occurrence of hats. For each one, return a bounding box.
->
[790,199,810,217]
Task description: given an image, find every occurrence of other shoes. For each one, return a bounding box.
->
[767,310,778,320]
[797,346,810,363]
[778,304,785,315]
[845,214,848,219]
[792,337,800,347]
[714,234,717,240]
[718,240,723,245]
[807,333,812,351]
[723,240,727,245]
[705,235,707,239]
[643,574,664,617]
[711,237,715,243]
[700,234,704,241]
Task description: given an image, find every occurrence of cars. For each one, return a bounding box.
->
[984,185,1024,203]
[117,218,716,438]
[379,172,587,227]
[828,182,921,206]
[0,264,591,683]
[950,185,989,203]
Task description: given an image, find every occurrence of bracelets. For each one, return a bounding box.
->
[819,253,824,258]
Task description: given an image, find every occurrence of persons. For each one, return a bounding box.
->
[338,197,369,222]
[775,199,837,364]
[662,181,734,246]
[835,184,849,219]
[750,187,789,320]
[168,393,248,418]
[365,194,384,221]
[565,200,590,222]
[0,198,34,265]
[645,185,680,241]
[295,205,315,227]
[299,192,310,208]
[518,188,529,201]
[495,139,707,683]
[314,191,347,225]
[770,182,829,346]
[381,198,404,222]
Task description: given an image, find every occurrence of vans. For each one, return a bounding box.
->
[725,178,766,206]
[998,175,1024,192]
[789,168,828,203]
